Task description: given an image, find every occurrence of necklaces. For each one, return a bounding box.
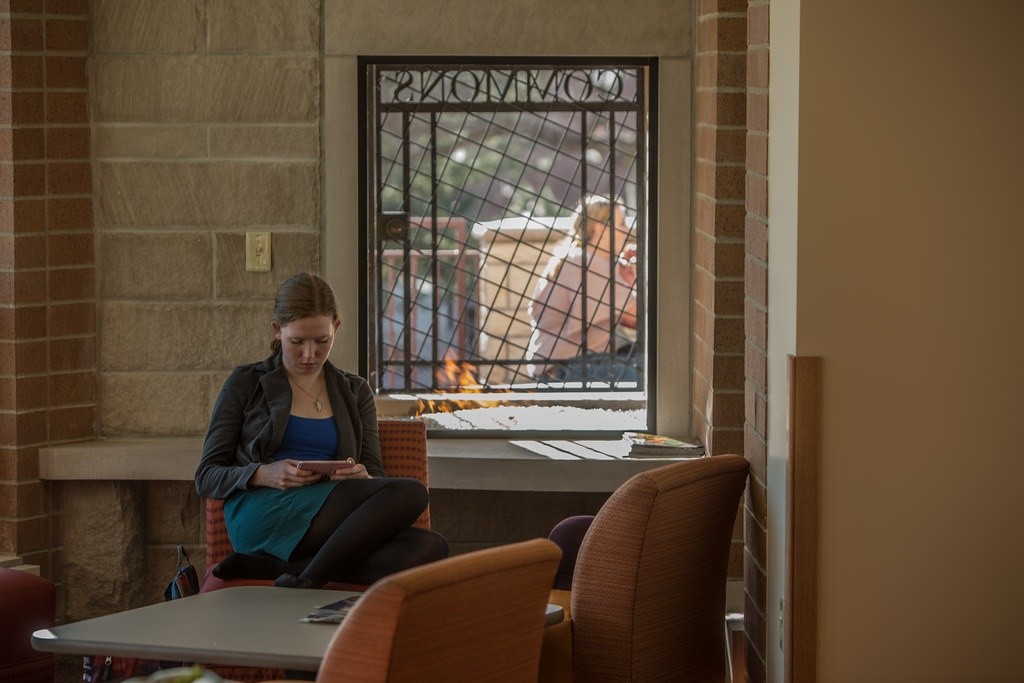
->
[287,374,326,413]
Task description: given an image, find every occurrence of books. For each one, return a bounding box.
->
[300,595,362,623]
[622,433,705,458]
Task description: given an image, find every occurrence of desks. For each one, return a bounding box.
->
[31,584,564,683]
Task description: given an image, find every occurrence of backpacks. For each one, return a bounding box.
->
[83,656,160,683]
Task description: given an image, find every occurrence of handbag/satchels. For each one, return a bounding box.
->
[164,546,200,601]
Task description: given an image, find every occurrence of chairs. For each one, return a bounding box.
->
[261,537,563,683]
[197,417,428,682]
[548,454,750,683]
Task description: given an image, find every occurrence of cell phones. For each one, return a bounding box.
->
[296,461,354,478]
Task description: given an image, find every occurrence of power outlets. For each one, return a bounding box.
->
[245,231,271,272]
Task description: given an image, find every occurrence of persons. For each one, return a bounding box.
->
[526,196,638,382]
[196,272,449,589]
